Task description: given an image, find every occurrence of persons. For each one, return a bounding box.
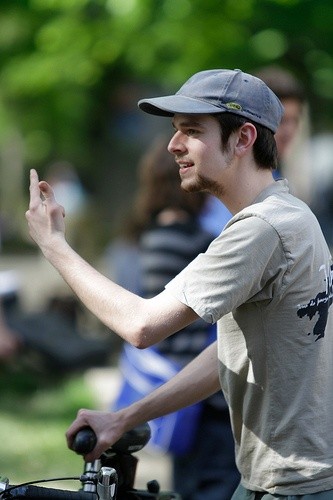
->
[1,157,142,391]
[77,124,241,500]
[197,68,306,239]
[25,69,332,500]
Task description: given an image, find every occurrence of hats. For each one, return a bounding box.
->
[137,68,285,135]
